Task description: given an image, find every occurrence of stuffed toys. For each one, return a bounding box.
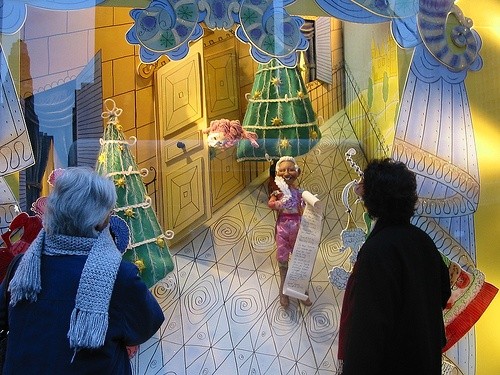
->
[203,120,259,150]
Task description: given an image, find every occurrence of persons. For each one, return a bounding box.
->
[0,165,165,375]
[269,155,318,309]
[338,157,451,375]
